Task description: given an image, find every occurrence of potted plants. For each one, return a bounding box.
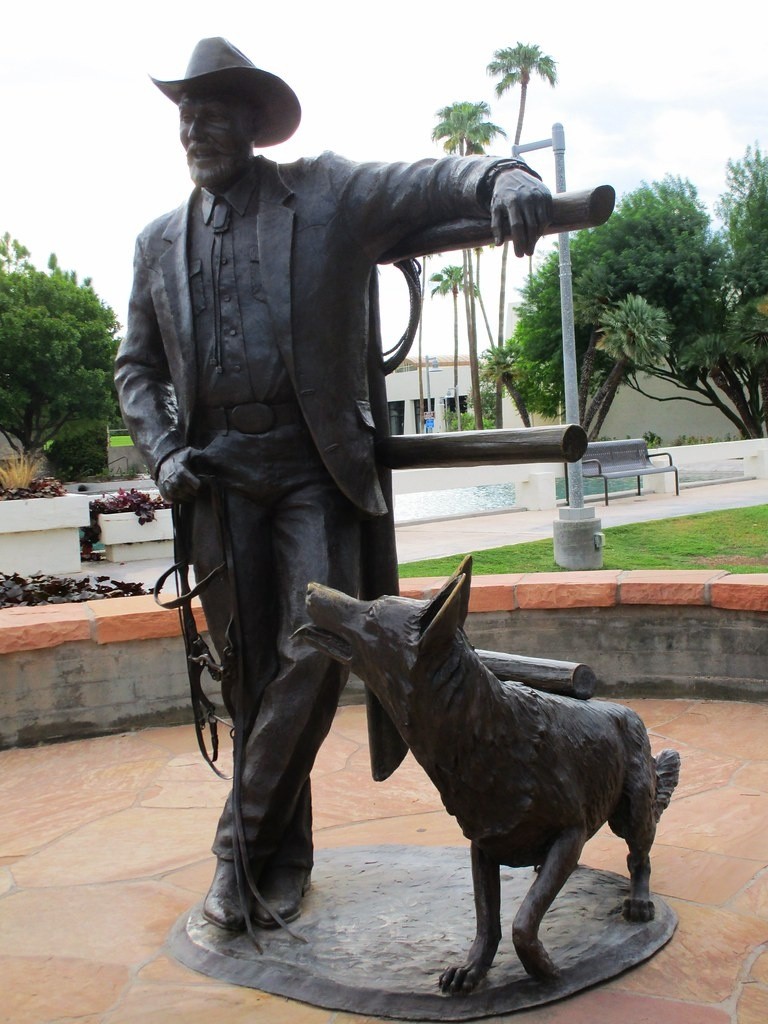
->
[0,448,92,575]
[81,488,175,563]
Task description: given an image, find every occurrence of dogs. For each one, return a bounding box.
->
[299,555,681,994]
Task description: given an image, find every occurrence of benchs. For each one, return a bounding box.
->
[583,439,679,506]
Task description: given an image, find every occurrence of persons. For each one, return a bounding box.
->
[113,36,554,934]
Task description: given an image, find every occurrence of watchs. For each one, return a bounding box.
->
[485,160,531,196]
